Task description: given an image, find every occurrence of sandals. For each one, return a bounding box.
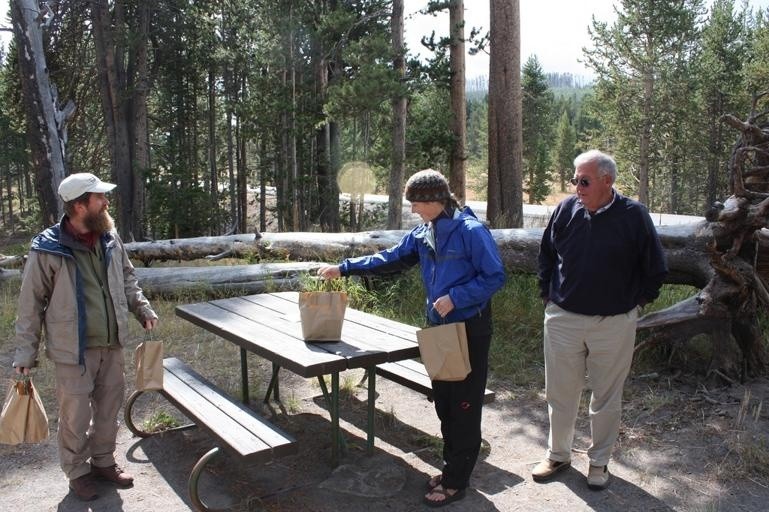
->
[426,475,442,490]
[424,484,466,507]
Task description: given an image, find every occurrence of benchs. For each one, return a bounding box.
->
[355,359,497,406]
[123,356,299,510]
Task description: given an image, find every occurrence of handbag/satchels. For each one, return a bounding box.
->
[0,374,49,446]
[299,275,348,342]
[136,328,165,392]
[416,306,473,382]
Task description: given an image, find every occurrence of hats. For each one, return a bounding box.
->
[58,173,117,202]
[405,168,451,202]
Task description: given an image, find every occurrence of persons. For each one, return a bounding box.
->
[316,169,506,507]
[14,172,158,501]
[533,148,670,491]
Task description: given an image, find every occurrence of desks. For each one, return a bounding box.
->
[173,290,428,471]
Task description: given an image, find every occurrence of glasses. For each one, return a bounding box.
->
[570,175,606,187]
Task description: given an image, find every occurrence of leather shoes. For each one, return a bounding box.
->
[68,475,97,500]
[532,458,571,481]
[90,462,134,486]
[587,464,609,490]
